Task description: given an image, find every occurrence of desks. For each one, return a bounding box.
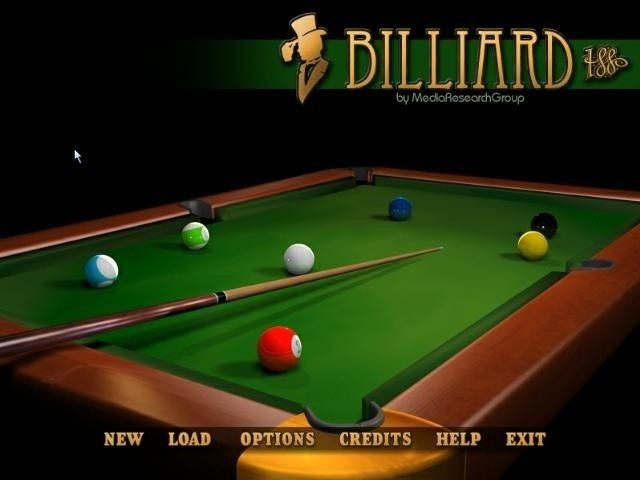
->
[1,165,638,480]
